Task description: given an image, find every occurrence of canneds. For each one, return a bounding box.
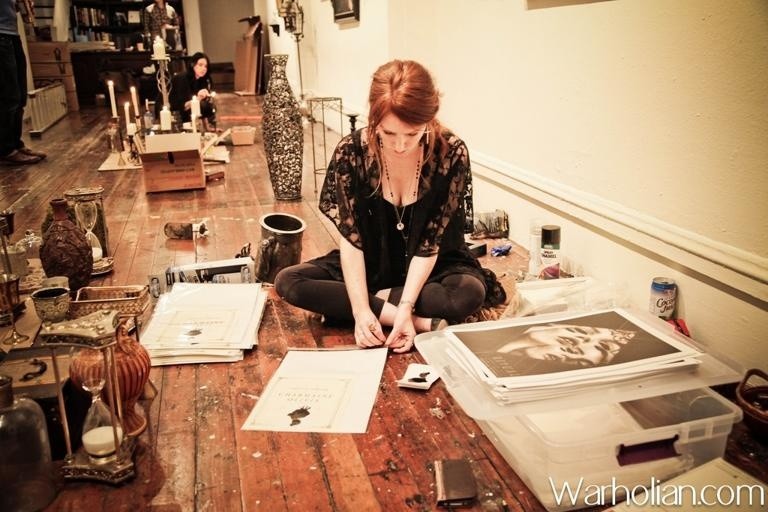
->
[647,276,677,323]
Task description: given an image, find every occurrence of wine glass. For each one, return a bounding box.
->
[0,271,30,346]
[0,209,16,247]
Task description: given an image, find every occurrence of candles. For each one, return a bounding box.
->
[106,39,201,134]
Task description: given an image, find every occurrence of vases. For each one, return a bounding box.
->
[30,196,95,325]
[260,52,305,202]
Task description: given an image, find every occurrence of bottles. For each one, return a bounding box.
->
[527,223,561,281]
[0,372,58,512]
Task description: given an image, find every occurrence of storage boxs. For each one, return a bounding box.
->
[413,328,744,512]
[136,132,207,195]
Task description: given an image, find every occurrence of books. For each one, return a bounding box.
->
[433,302,702,405]
[141,279,271,370]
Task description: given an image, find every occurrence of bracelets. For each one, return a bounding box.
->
[397,300,417,314]
[378,138,423,235]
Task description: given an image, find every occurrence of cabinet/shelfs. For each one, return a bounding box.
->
[26,40,80,112]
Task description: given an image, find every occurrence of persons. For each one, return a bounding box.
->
[0,0,47,165]
[155,52,223,133]
[141,1,183,51]
[274,56,509,355]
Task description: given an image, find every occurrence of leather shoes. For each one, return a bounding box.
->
[1,149,45,165]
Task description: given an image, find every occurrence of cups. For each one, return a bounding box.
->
[29,286,70,324]
[41,275,69,290]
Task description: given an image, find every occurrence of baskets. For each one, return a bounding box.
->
[736,369,768,429]
